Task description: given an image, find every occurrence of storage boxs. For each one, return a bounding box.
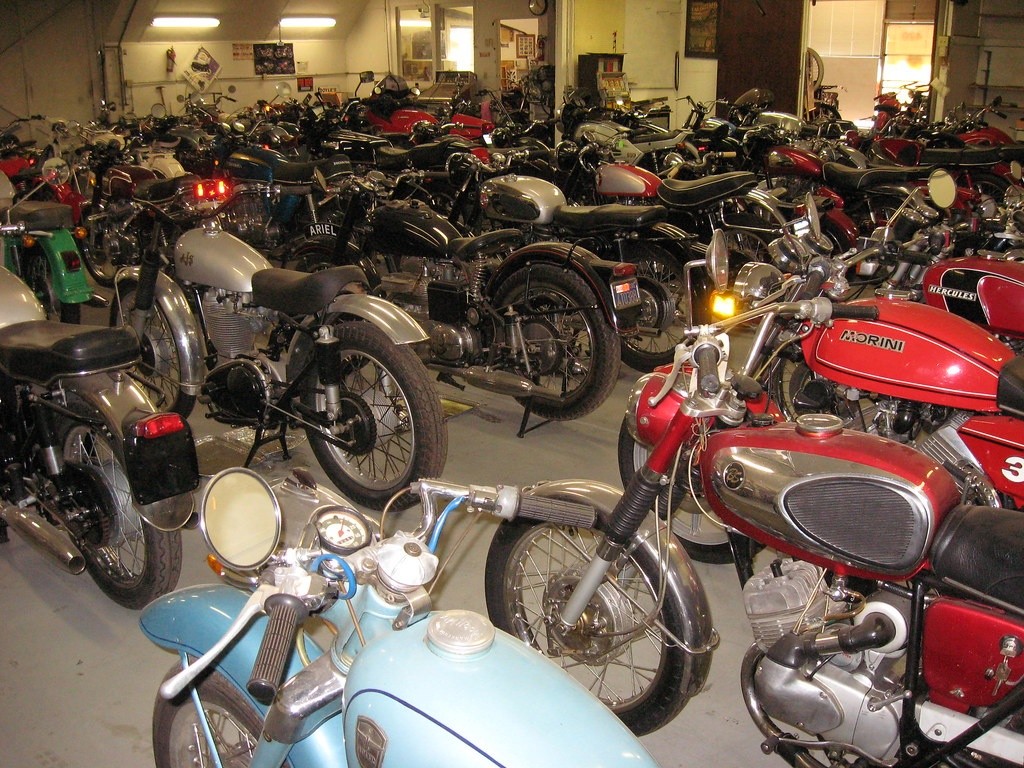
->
[597,72,631,110]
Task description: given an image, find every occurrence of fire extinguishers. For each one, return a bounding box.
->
[166,46,177,71]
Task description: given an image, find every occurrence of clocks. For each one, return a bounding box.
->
[528,0,548,16]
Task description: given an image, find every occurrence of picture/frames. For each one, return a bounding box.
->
[516,34,536,58]
[684,0,720,59]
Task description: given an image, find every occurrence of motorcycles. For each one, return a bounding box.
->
[0,73,1023,768]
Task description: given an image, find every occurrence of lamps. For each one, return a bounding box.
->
[277,16,336,28]
[148,14,220,27]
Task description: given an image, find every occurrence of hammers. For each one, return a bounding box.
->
[157,86,166,105]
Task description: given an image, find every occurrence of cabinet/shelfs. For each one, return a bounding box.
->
[577,54,624,106]
[967,82,1024,125]
[501,59,530,92]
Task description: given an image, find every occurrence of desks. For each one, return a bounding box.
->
[627,111,670,131]
